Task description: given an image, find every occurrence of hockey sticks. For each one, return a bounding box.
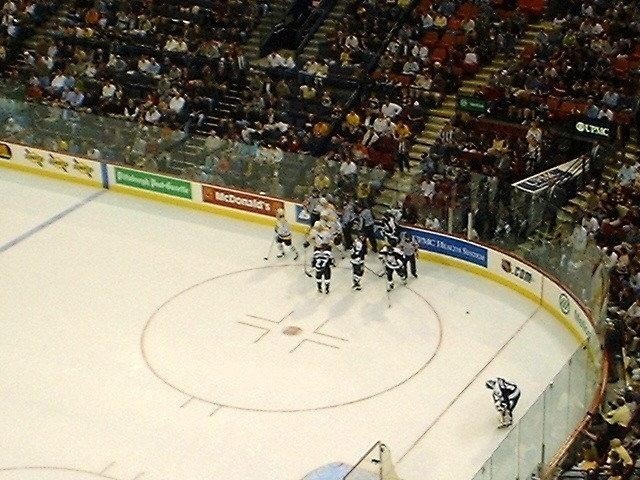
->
[304,250,312,277]
[365,265,385,277]
[264,240,274,260]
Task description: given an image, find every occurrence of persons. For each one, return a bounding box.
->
[379,237,410,293]
[0,0,640,273]
[311,242,338,294]
[350,241,366,292]
[401,233,420,279]
[552,273,640,480]
[486,377,522,429]
